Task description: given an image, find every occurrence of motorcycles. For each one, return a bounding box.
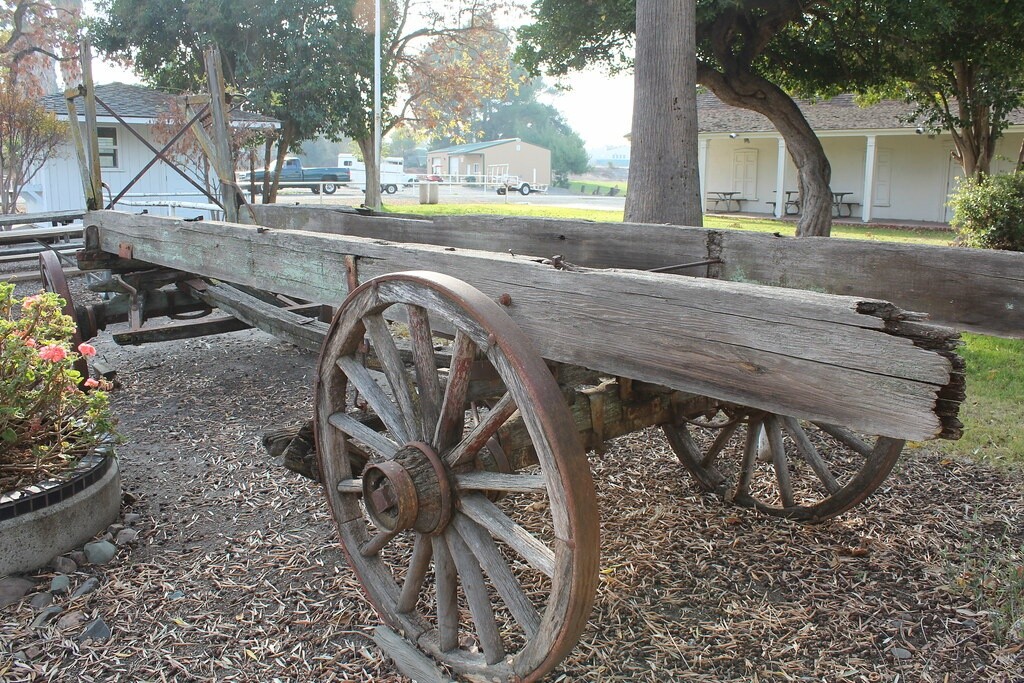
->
[496,177,530,196]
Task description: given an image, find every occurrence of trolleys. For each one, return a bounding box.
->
[39,45,1023,683]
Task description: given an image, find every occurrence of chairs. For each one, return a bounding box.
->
[706,189,861,220]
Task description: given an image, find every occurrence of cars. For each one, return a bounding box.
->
[426,175,443,182]
[403,172,419,187]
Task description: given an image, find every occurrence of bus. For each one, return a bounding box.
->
[338,154,404,195]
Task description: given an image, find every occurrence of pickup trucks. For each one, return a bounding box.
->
[239,157,350,196]
